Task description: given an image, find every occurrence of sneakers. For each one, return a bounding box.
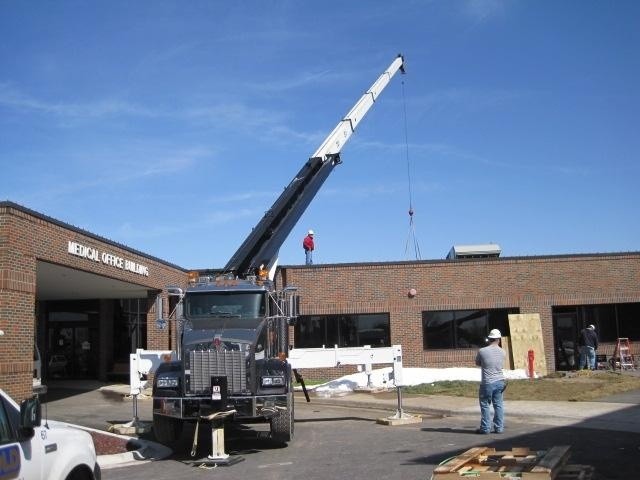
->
[476,428,504,434]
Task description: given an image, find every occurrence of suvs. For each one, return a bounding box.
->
[0,389,105,480]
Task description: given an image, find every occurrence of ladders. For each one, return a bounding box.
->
[612,338,635,374]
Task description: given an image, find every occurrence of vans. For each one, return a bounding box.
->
[32,343,48,398]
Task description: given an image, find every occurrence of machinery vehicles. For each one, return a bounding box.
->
[105,50,427,473]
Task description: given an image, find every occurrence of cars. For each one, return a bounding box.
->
[48,352,70,371]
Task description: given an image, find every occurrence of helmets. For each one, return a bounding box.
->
[308,230,314,235]
[586,324,595,330]
[488,328,502,339]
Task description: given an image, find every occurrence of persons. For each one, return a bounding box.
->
[576,324,598,370]
[303,230,314,265]
[476,329,506,434]
